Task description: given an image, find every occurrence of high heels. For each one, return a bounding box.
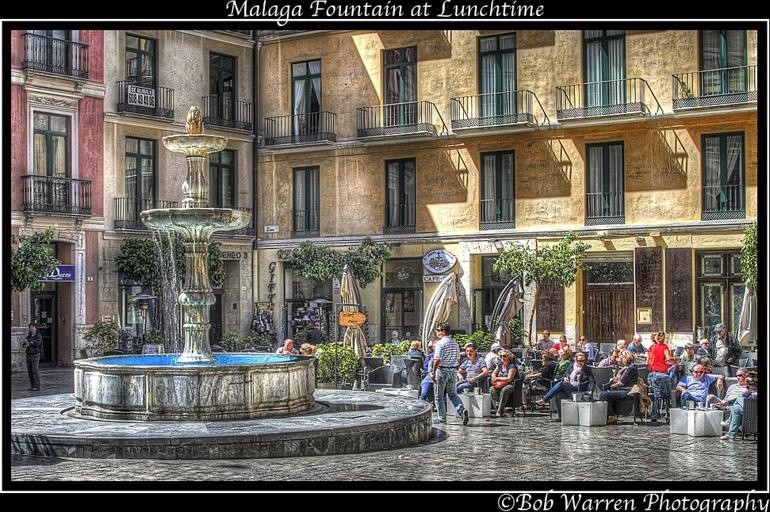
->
[608,417,618,425]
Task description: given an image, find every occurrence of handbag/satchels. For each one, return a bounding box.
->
[491,377,509,391]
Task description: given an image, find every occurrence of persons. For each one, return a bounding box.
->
[403,322,757,441]
[387,330,401,346]
[275,339,318,358]
[22,321,44,391]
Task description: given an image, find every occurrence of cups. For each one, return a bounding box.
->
[464,387,482,394]
[685,399,705,411]
[710,403,715,408]
[592,360,609,368]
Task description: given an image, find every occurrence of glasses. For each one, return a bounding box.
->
[577,359,586,362]
[735,374,745,377]
[436,327,446,332]
[500,354,509,358]
[693,369,704,374]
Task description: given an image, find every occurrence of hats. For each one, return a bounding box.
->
[712,323,724,332]
[491,342,501,350]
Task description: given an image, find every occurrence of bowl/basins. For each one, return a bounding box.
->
[573,392,593,402]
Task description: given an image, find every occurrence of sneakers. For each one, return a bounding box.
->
[719,433,735,441]
[720,419,730,428]
[432,417,447,424]
[534,399,546,407]
[460,408,470,425]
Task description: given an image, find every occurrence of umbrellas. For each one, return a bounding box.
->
[340,264,367,380]
[420,271,460,356]
[736,273,757,346]
[486,271,525,344]
